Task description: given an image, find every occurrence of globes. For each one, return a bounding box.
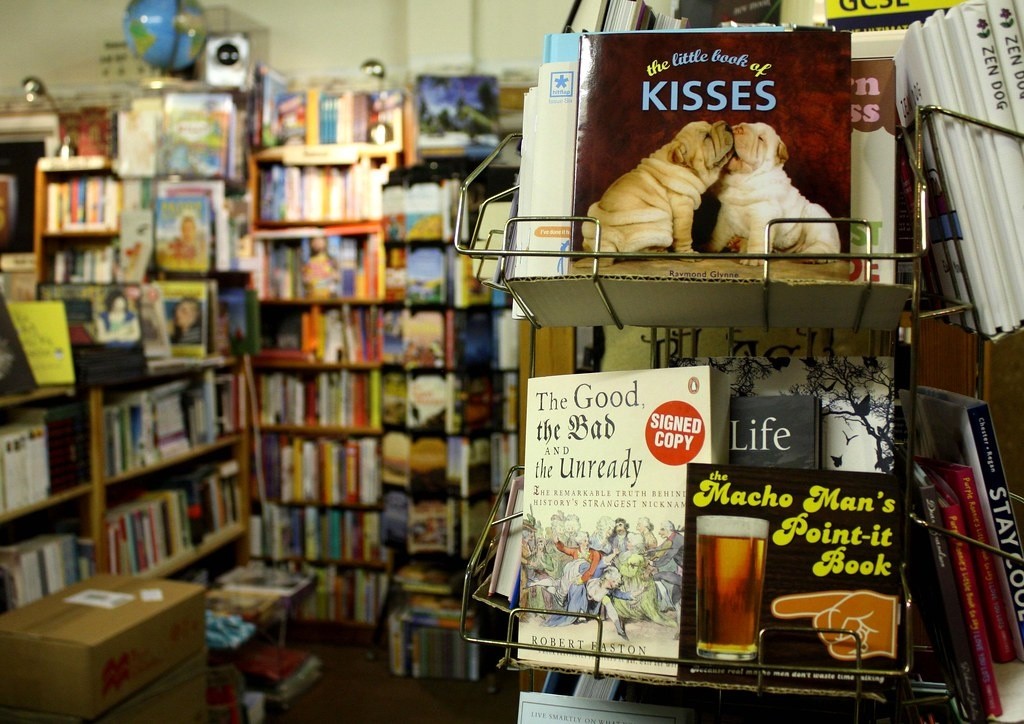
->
[121,0,209,90]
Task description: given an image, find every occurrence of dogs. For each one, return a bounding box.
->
[572,118,843,268]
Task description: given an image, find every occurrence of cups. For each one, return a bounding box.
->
[697,515,773,662]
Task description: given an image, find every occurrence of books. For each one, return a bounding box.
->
[0,0,1024,724]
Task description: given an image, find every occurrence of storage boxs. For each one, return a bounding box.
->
[0,644,205,724]
[0,576,205,724]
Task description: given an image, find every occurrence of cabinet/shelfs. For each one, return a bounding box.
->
[0,0,1024,724]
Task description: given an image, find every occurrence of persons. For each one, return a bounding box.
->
[180,213,199,257]
[170,297,202,345]
[96,289,141,344]
